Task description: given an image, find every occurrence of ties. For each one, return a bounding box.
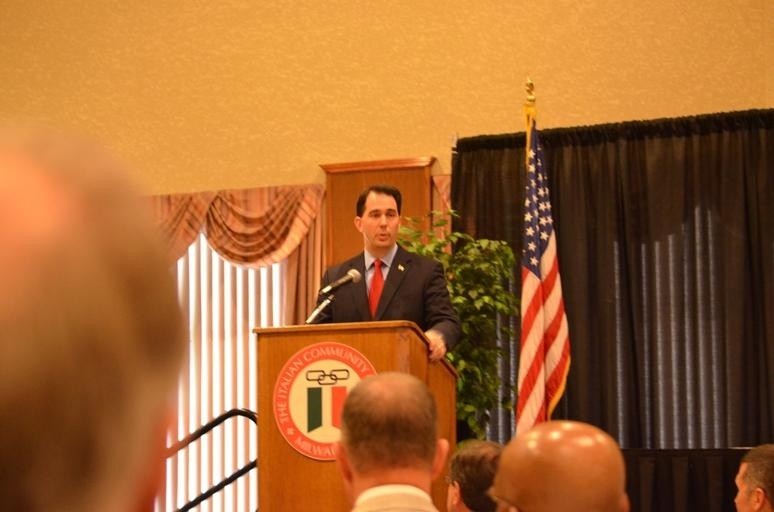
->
[366,258,386,320]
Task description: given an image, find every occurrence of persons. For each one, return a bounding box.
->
[310,185,463,365]
[445,439,504,511]
[734,444,773,511]
[493,418,633,512]
[333,368,449,511]
[0,139,193,508]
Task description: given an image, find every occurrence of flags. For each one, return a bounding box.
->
[509,105,573,439]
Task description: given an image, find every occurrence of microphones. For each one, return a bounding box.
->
[319,268,362,296]
